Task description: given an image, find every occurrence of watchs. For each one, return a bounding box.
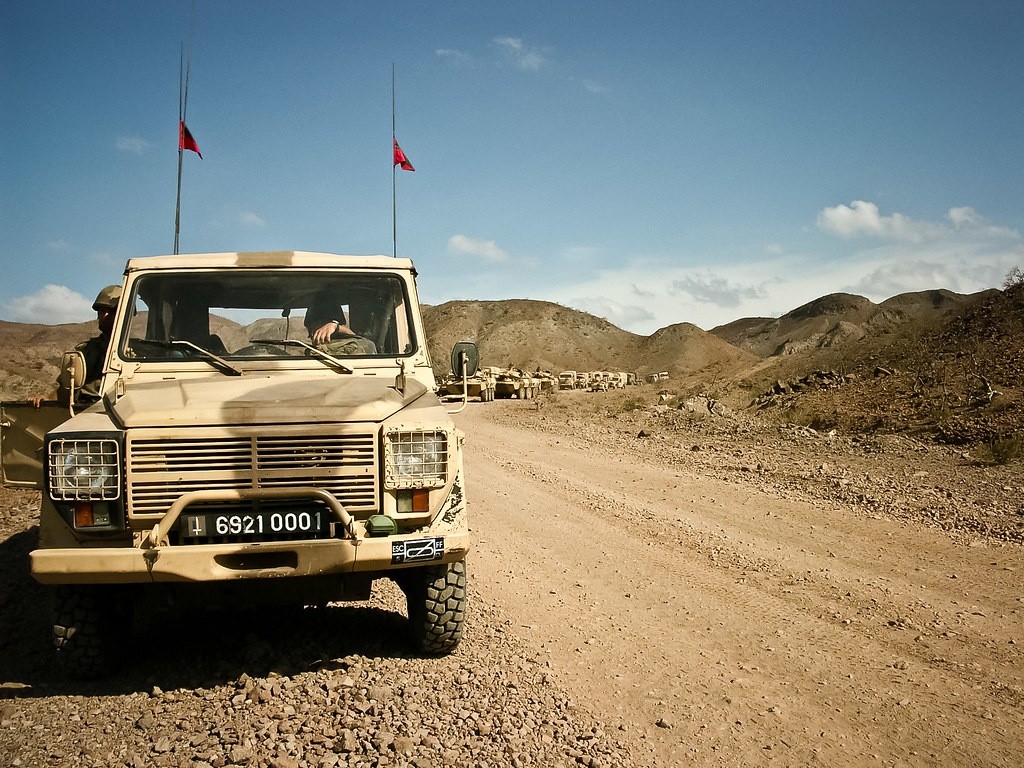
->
[330,320,339,333]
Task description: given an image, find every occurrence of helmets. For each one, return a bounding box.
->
[303,300,347,325]
[92,285,137,316]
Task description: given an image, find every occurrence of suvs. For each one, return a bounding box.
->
[0,251,480,688]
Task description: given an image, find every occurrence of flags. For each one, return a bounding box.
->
[179,120,203,160]
[393,139,415,171]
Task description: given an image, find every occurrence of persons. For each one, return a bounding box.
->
[304,295,355,344]
[537,366,540,372]
[27,285,136,408]
